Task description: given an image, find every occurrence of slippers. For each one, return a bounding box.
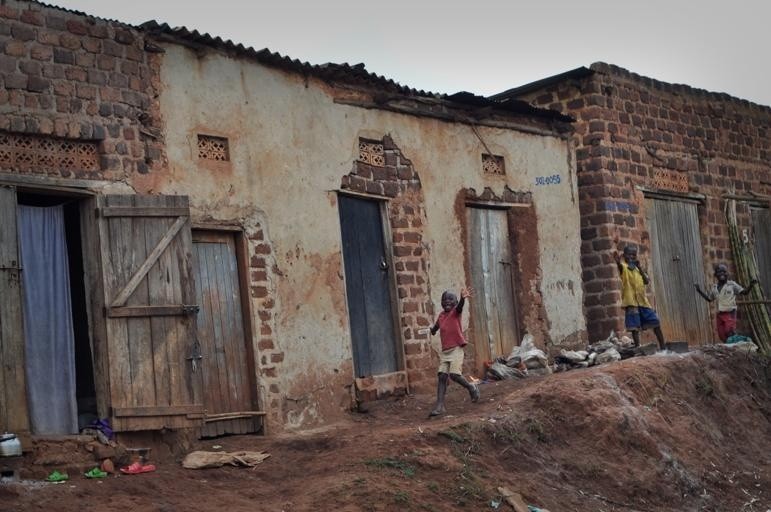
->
[469,382,480,402]
[429,409,447,416]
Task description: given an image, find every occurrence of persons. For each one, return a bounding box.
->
[613,245,667,353]
[694,264,759,342]
[431,286,480,416]
